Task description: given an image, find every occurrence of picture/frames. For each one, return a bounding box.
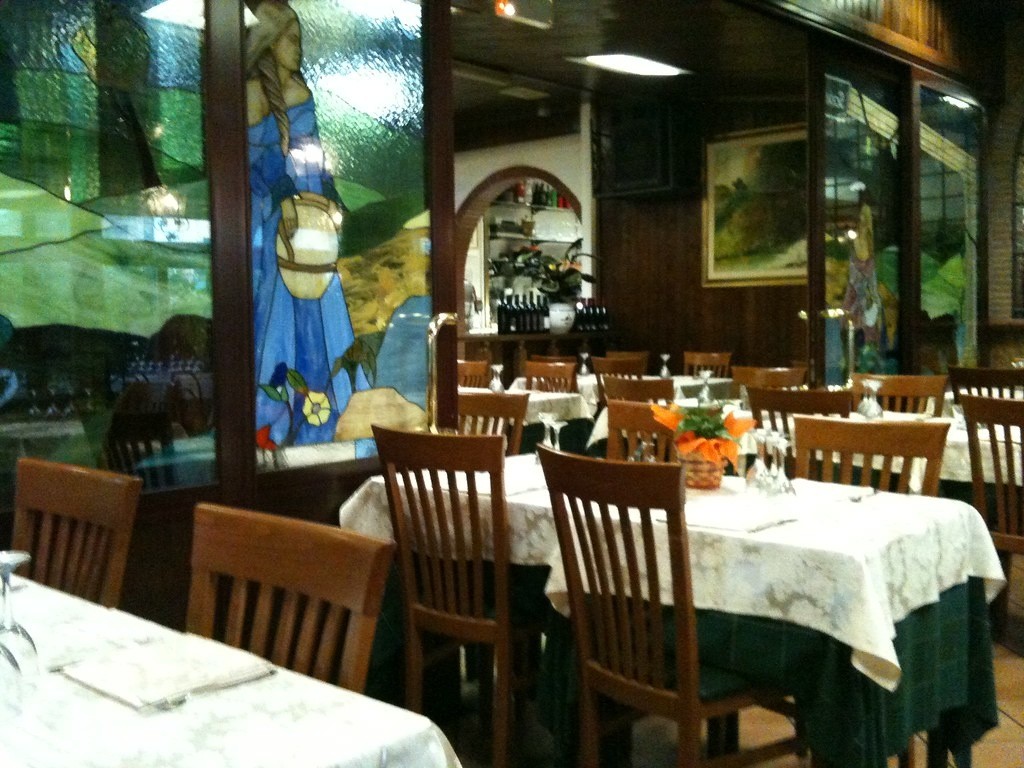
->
[700,120,807,287]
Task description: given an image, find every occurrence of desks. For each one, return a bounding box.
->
[457,386,582,453]
[601,399,1024,528]
[0,421,94,507]
[0,574,433,768]
[516,375,732,414]
[368,452,975,768]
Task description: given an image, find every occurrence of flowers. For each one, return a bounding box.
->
[513,238,605,302]
[652,397,758,471]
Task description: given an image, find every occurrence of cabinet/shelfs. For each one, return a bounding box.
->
[489,200,574,244]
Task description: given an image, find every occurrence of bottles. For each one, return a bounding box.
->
[533,182,552,206]
[528,291,538,333]
[506,295,516,334]
[536,295,544,332]
[552,187,571,208]
[522,294,531,332]
[573,298,608,331]
[542,297,550,334]
[497,295,508,335]
[514,294,525,334]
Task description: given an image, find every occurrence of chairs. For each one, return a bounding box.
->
[458,391,531,452]
[79,410,178,487]
[370,422,575,768]
[591,356,643,408]
[792,418,952,495]
[732,367,807,391]
[525,361,578,394]
[607,350,650,375]
[957,394,1024,650]
[852,373,949,419]
[946,364,1024,429]
[748,389,853,478]
[684,351,733,380]
[607,398,723,464]
[187,503,400,693]
[11,458,144,610]
[535,442,807,768]
[458,361,492,388]
[533,355,579,394]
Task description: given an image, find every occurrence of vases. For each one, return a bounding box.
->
[677,452,728,491]
[548,302,576,334]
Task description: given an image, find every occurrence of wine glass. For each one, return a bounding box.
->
[27,383,94,420]
[0,550,40,715]
[489,365,505,394]
[660,354,671,378]
[580,353,589,375]
[697,370,715,406]
[535,412,566,464]
[745,429,798,504]
[858,379,882,419]
